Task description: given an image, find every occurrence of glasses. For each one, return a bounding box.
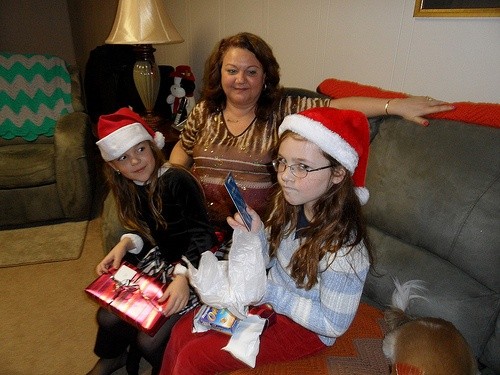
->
[272,156,335,178]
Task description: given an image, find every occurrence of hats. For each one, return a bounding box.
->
[95,107,166,162]
[278,107,370,207]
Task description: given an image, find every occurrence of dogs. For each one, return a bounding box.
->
[381,275,483,375]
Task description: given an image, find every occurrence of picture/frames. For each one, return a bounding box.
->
[412,0,500,19]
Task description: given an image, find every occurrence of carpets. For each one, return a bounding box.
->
[0,220,88,267]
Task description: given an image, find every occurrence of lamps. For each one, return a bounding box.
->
[104,0,186,132]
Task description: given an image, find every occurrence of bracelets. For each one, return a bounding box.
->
[385,100,390,115]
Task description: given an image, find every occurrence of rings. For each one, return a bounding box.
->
[427,96,432,101]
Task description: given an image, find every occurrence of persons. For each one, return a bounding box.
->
[89,111,220,375]
[166,31,456,253]
[157,107,370,375]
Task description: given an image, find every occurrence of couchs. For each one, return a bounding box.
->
[101,79,500,375]
[0,50,98,229]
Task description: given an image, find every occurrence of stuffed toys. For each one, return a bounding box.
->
[167,65,196,120]
[382,306,480,375]
